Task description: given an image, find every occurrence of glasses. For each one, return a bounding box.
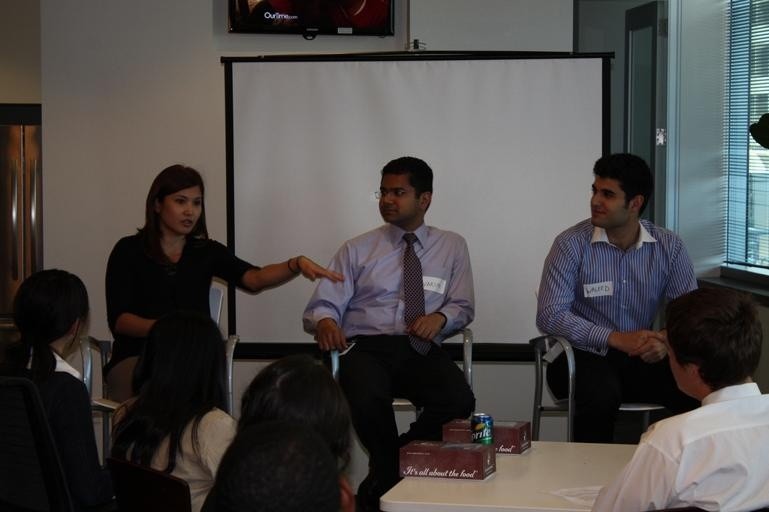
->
[374,189,422,199]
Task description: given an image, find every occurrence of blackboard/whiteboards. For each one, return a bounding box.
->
[407,0,579,54]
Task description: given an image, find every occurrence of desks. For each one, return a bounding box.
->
[372,437,644,510]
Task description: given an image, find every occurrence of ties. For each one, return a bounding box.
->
[399,231,436,359]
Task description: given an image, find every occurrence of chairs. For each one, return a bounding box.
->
[69,333,123,415]
[102,468,193,511]
[319,322,477,432]
[94,284,242,464]
[1,371,86,510]
[527,286,667,443]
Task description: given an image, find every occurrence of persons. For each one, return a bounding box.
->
[535,153,703,444]
[107,309,239,512]
[301,156,476,511]
[103,166,345,406]
[200,353,355,512]
[590,286,769,512]
[0,268,107,512]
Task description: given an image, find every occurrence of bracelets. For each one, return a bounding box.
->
[296,255,304,271]
[288,259,299,274]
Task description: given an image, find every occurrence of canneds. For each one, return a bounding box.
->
[472,412,493,445]
